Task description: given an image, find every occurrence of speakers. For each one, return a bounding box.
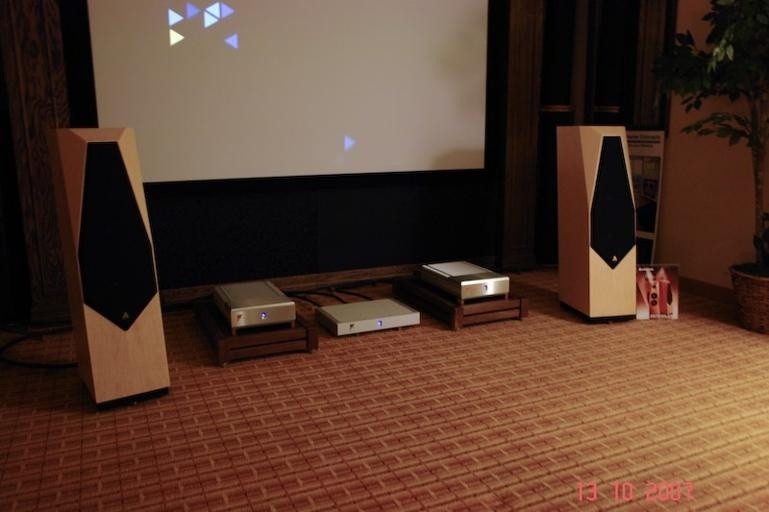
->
[556,124,638,322]
[46,123,172,412]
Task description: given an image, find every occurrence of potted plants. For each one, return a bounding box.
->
[652,1,769,334]
